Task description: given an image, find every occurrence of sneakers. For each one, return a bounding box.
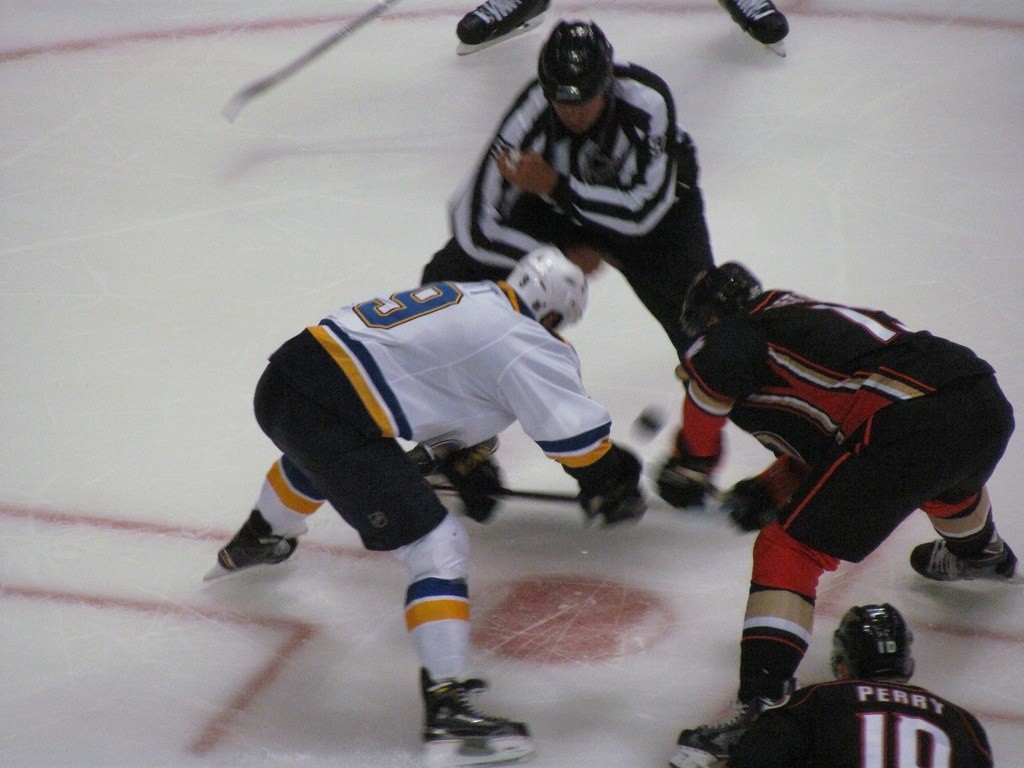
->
[909,523,1023,590]
[455,0,551,55]
[717,0,789,57]
[420,666,535,767]
[202,509,297,581]
[669,677,797,768]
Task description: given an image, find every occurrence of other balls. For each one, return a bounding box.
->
[639,411,661,431]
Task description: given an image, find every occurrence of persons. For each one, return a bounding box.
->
[716,599,999,768]
[417,15,723,372]
[656,260,1019,767]
[214,241,647,764]
[453,0,792,59]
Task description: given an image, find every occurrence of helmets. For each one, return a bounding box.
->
[504,247,588,331]
[832,604,909,679]
[680,263,761,339]
[539,22,614,104]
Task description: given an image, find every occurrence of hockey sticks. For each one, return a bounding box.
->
[223,1,395,123]
[676,467,735,503]
[412,483,613,502]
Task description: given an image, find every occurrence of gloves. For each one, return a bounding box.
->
[725,479,780,531]
[562,445,648,522]
[438,447,503,522]
[656,433,718,508]
